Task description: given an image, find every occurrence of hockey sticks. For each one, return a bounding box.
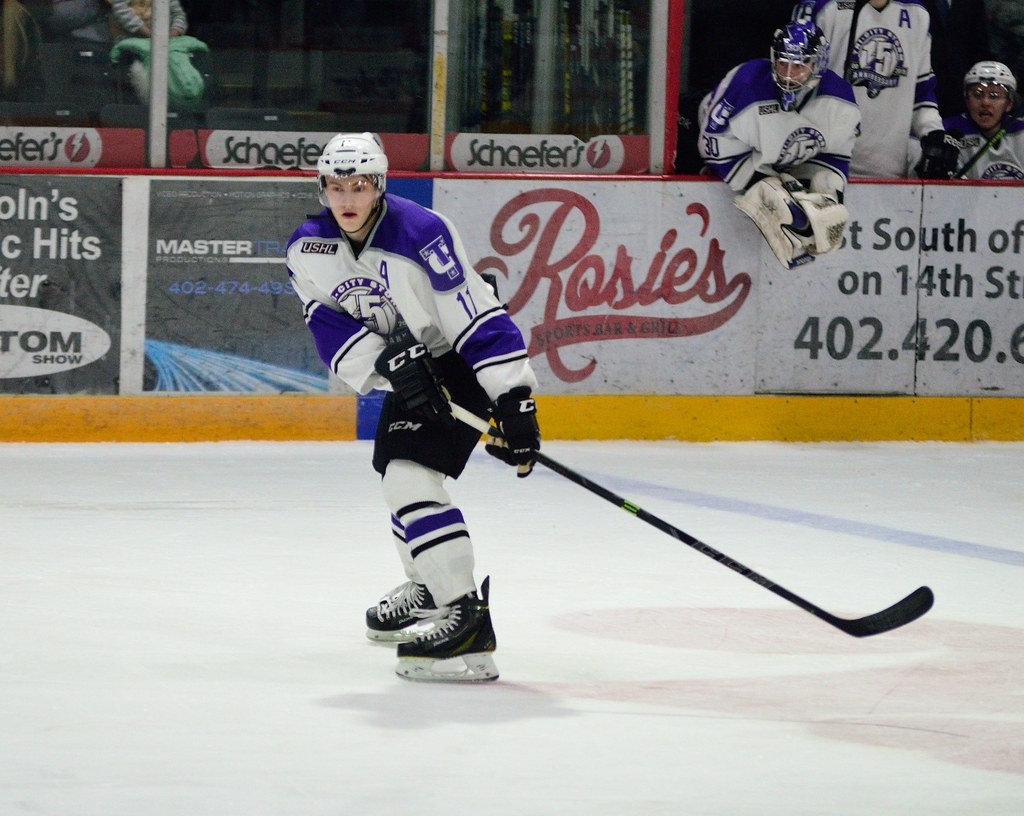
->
[449,400,936,639]
[460,0,637,136]
[843,0,870,85]
[956,108,1023,180]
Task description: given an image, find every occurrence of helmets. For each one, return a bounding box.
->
[770,19,827,92]
[317,132,388,191]
[963,61,1017,98]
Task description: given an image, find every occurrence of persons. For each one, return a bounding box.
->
[794,0,960,180]
[944,59,1024,180]
[698,17,862,270]
[284,130,540,685]
[110,0,189,108]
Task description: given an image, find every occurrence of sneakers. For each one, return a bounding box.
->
[365,581,438,641]
[395,574,499,680]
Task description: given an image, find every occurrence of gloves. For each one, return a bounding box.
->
[485,386,541,478]
[913,129,961,180]
[374,343,456,427]
